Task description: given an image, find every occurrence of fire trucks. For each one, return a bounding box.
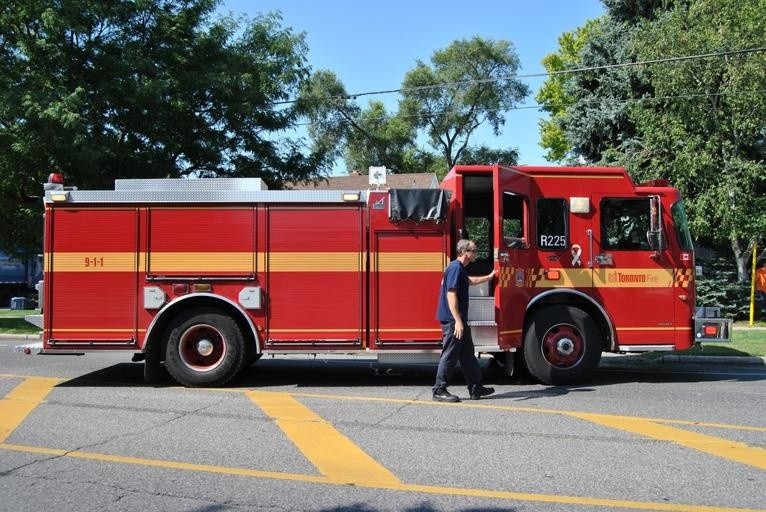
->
[9,156,736,394]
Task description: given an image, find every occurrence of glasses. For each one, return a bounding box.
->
[466,249,478,253]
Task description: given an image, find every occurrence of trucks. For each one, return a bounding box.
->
[0,251,43,304]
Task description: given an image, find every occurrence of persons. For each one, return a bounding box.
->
[432,239,499,402]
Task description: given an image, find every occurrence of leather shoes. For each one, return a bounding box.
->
[470,387,495,400]
[433,390,459,402]
